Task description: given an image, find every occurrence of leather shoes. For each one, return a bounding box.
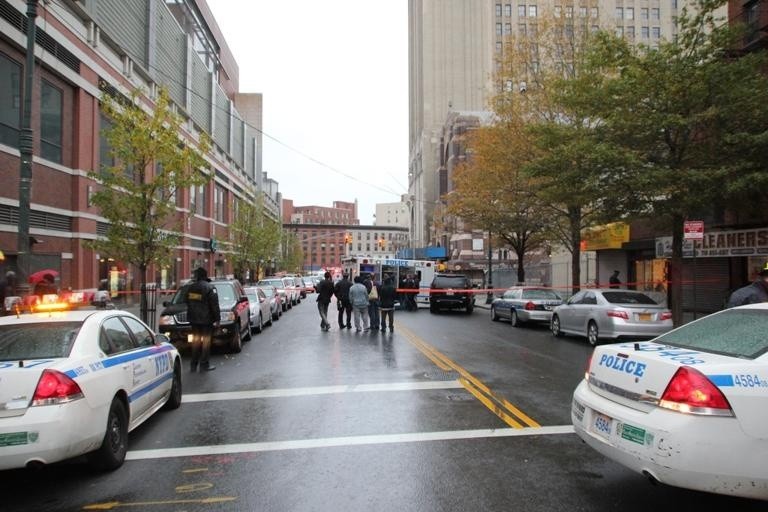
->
[199,365,216,372]
[190,366,197,373]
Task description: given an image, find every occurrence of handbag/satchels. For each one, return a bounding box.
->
[367,281,379,300]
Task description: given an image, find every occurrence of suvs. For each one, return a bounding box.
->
[158,274,256,355]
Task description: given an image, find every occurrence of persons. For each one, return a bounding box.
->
[726,261,768,309]
[182,267,221,371]
[40,274,57,294]
[314,269,419,333]
[609,270,621,289]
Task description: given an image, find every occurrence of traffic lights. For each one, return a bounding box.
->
[382,240,387,247]
[348,235,352,244]
[378,240,382,247]
[344,234,348,244]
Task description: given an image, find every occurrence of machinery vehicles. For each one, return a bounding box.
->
[336,254,434,313]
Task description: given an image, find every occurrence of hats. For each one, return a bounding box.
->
[193,267,208,278]
[43,274,56,283]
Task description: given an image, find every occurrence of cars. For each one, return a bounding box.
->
[564,295,767,507]
[242,272,323,335]
[487,284,566,329]
[548,285,677,348]
[0,283,187,487]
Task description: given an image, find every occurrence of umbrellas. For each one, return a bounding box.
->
[24,269,59,284]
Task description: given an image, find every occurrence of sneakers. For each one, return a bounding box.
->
[320,324,394,334]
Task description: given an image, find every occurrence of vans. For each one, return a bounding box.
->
[427,270,477,315]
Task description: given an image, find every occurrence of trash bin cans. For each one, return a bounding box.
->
[145,282,158,335]
